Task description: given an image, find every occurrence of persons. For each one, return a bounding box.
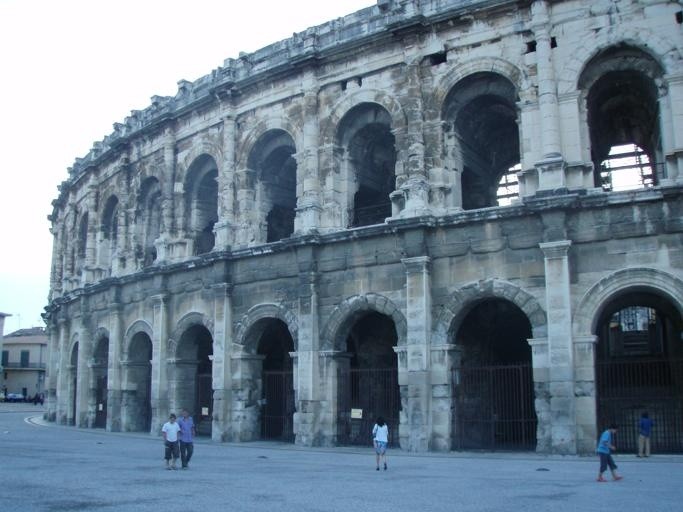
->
[161,409,196,471]
[635,411,656,458]
[371,416,389,471]
[6,390,45,406]
[595,423,622,482]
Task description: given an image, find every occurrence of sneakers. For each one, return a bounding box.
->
[597,475,626,483]
[376,463,387,470]
[167,465,188,470]
